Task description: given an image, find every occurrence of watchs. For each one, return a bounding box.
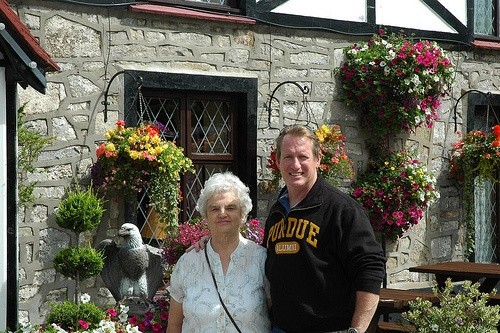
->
[348,328,358,333]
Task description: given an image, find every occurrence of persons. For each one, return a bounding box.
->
[185,124,386,333]
[165,172,272,333]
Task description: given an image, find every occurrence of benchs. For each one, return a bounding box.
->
[378,322,416,333]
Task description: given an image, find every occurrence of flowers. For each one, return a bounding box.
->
[90,121,281,276]
[39,292,167,333]
[313,28,500,242]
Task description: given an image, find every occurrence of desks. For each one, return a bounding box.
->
[409,262,500,292]
[375,288,454,325]
